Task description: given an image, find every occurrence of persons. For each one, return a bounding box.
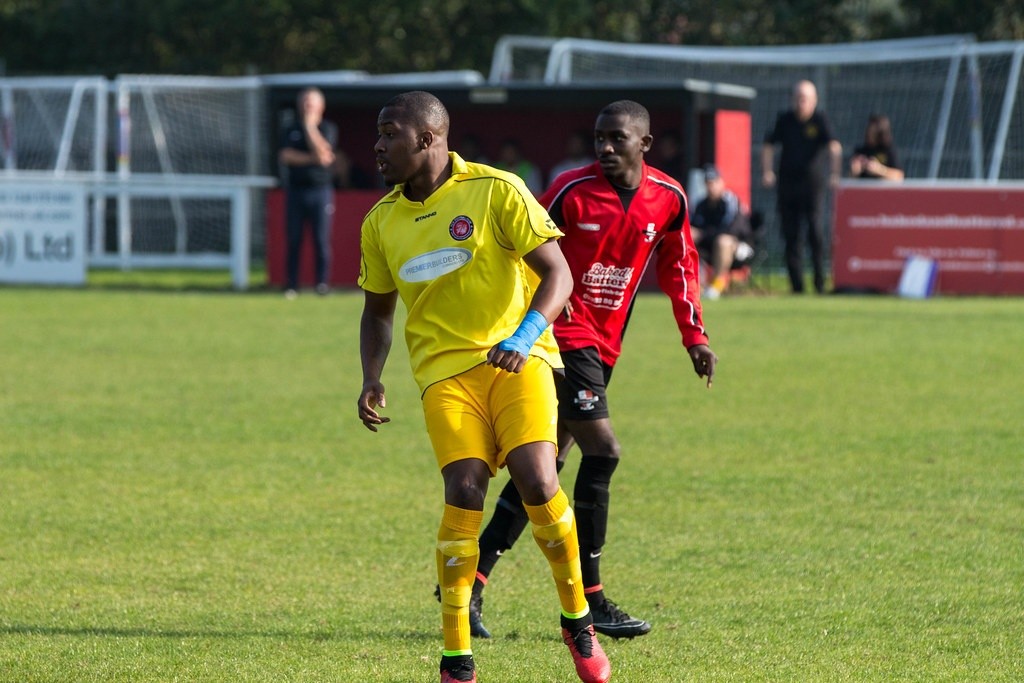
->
[277,87,339,296]
[690,167,757,302]
[456,133,489,164]
[494,136,544,195]
[434,100,718,641]
[646,125,683,180]
[545,129,595,186]
[763,79,842,296]
[357,93,615,683]
[849,114,904,184]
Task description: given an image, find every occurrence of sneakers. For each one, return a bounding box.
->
[590,591,652,642]
[434,577,491,639]
[440,656,477,683]
[560,610,611,683]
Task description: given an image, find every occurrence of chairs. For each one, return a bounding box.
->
[731,208,770,298]
